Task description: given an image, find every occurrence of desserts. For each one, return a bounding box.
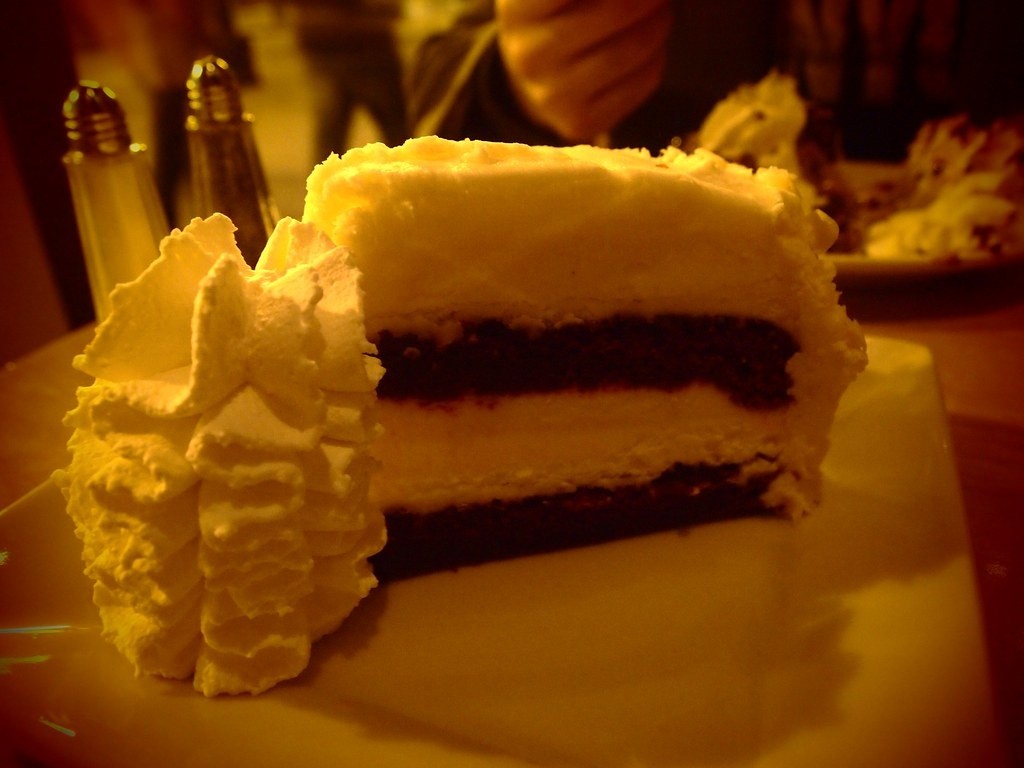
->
[665,73,1024,267]
[51,136,870,695]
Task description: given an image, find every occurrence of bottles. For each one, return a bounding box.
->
[61,79,171,326]
[184,54,281,270]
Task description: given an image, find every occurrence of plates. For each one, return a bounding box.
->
[0,319,1004,768]
[820,160,1024,289]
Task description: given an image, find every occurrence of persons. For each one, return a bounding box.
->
[405,0,1024,146]
[130,0,260,228]
[293,2,408,165]
